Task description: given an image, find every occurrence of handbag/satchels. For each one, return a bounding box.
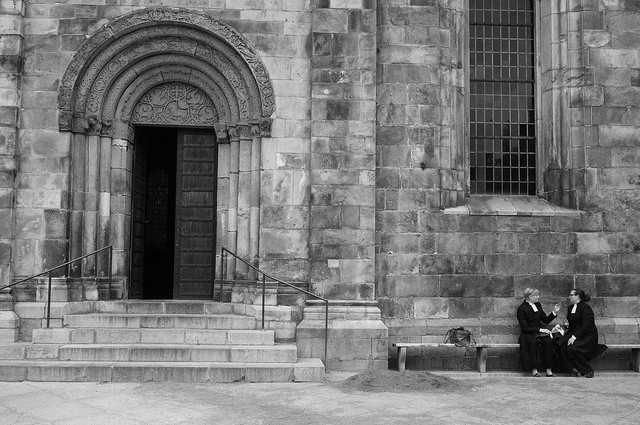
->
[444,326,471,347]
[535,320,552,344]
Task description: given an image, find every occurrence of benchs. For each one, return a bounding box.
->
[598,341,640,373]
[391,342,520,373]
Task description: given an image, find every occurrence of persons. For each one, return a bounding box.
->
[560,289,607,378]
[517,287,562,377]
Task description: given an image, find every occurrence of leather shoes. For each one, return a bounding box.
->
[575,372,582,377]
[586,371,594,378]
[533,373,541,377]
[546,374,553,377]
